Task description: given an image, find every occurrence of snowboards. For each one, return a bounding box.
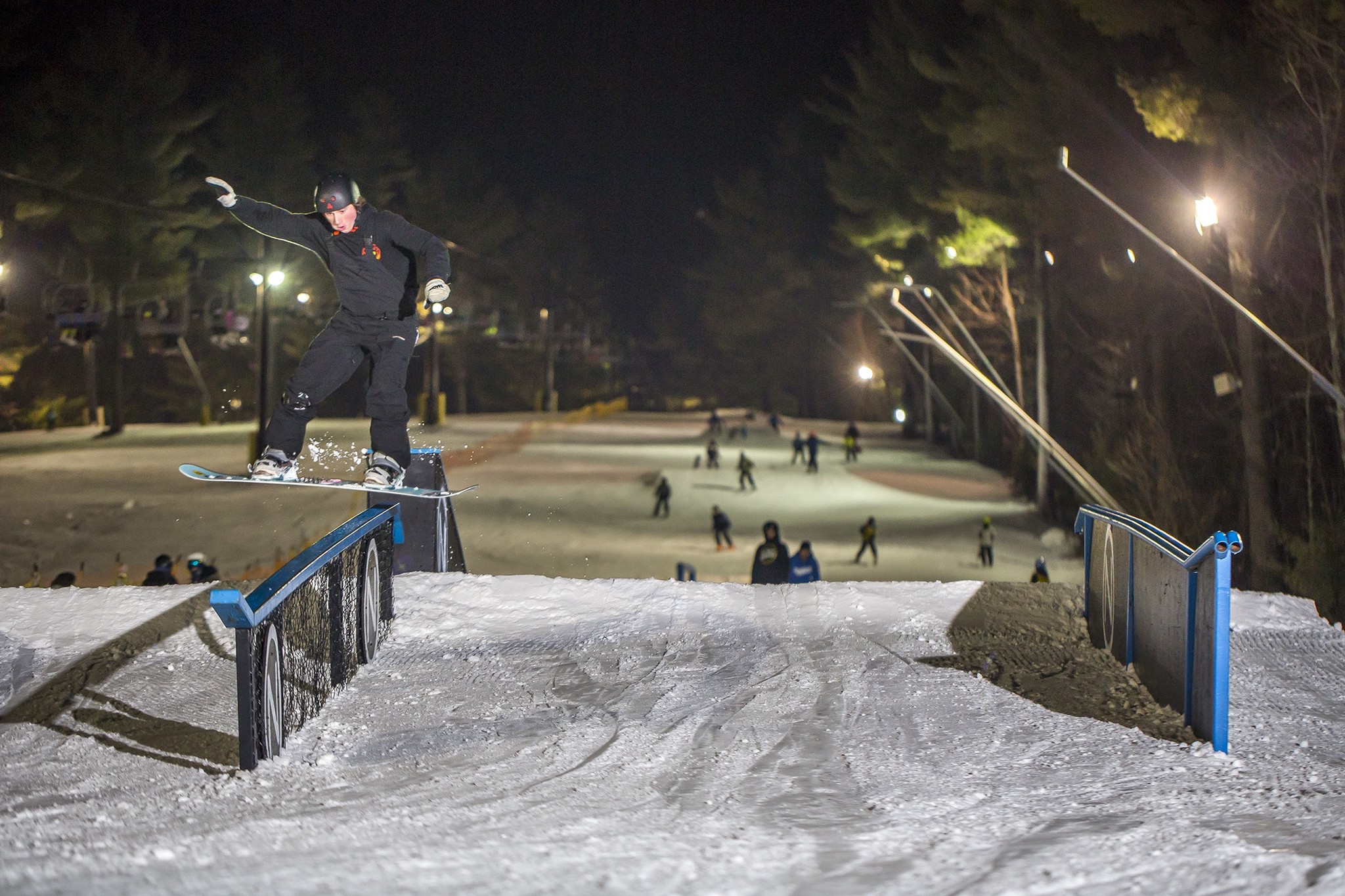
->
[179,463,478,497]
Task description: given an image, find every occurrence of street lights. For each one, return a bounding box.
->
[859,364,873,424]
[257,270,285,458]
[1194,194,1234,319]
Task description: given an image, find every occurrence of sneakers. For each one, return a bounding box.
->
[252,446,291,479]
[364,452,406,488]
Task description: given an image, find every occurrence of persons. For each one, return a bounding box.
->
[651,409,1050,586]
[206,170,451,491]
[187,551,220,584]
[49,571,76,590]
[142,553,178,586]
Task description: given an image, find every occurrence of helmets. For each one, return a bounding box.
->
[188,552,206,566]
[314,169,360,212]
[156,554,172,569]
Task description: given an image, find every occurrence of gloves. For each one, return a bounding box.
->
[424,279,451,309]
[205,177,238,207]
